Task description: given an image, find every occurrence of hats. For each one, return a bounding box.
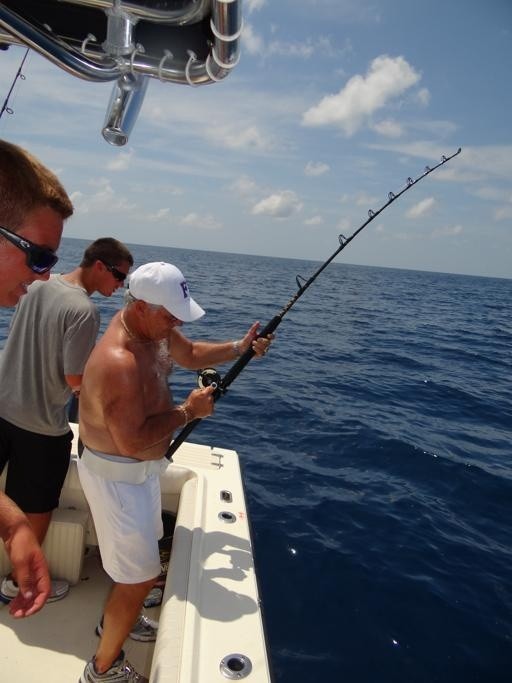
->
[128,261,205,323]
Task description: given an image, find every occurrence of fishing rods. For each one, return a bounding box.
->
[165,147,462,458]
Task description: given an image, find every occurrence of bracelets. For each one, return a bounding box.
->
[177,405,193,426]
[234,340,240,360]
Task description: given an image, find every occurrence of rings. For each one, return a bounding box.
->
[263,346,269,353]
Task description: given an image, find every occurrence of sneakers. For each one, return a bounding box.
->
[78,650,149,683]
[0,573,70,604]
[95,612,159,642]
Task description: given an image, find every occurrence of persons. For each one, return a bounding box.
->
[76,260,275,683]
[0,140,75,622]
[0,237,134,604]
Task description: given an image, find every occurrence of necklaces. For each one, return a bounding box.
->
[119,308,161,377]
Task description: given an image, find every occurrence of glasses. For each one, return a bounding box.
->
[111,268,126,281]
[0,226,58,275]
